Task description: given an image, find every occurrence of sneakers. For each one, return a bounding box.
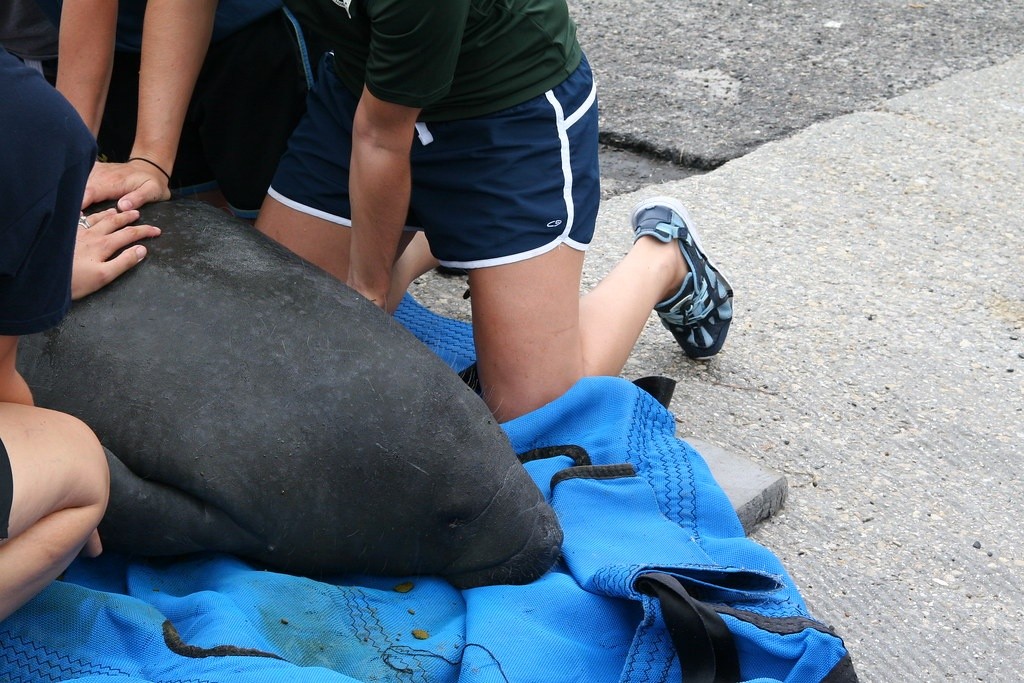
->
[630,196,734,361]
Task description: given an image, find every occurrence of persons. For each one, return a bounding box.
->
[0,1,732,625]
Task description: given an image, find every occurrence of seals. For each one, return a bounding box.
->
[15,142,565,592]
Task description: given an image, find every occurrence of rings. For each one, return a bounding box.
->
[78,216,91,229]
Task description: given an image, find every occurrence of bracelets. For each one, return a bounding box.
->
[128,158,171,181]
